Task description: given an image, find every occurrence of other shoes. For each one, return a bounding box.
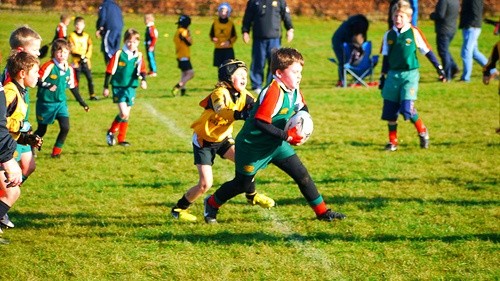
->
[52,154,60,159]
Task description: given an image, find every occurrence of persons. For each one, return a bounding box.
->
[429,0,459,81]
[0,24,42,187]
[95,0,124,66]
[203,46,346,223]
[482,23,500,136]
[172,14,194,97]
[209,2,238,83]
[102,28,147,146]
[48,14,71,45]
[332,14,369,87]
[0,79,23,188]
[241,0,294,95]
[0,52,44,234]
[388,1,418,31]
[378,0,447,151]
[29,38,90,158]
[67,16,101,101]
[455,0,500,82]
[349,34,373,67]
[143,13,158,76]
[170,59,275,222]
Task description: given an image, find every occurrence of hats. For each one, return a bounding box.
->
[218,59,247,81]
[176,15,190,25]
[218,3,230,17]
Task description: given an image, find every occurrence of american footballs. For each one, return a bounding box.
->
[284,110,314,146]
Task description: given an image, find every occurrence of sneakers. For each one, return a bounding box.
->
[418,129,429,148]
[385,143,397,151]
[120,142,129,146]
[90,97,97,100]
[317,208,346,222]
[171,205,197,222]
[107,131,115,146]
[248,194,275,208]
[204,198,218,224]
[172,87,178,96]
[148,73,157,76]
[0,213,14,227]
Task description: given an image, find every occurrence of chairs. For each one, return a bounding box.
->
[342,41,373,88]
